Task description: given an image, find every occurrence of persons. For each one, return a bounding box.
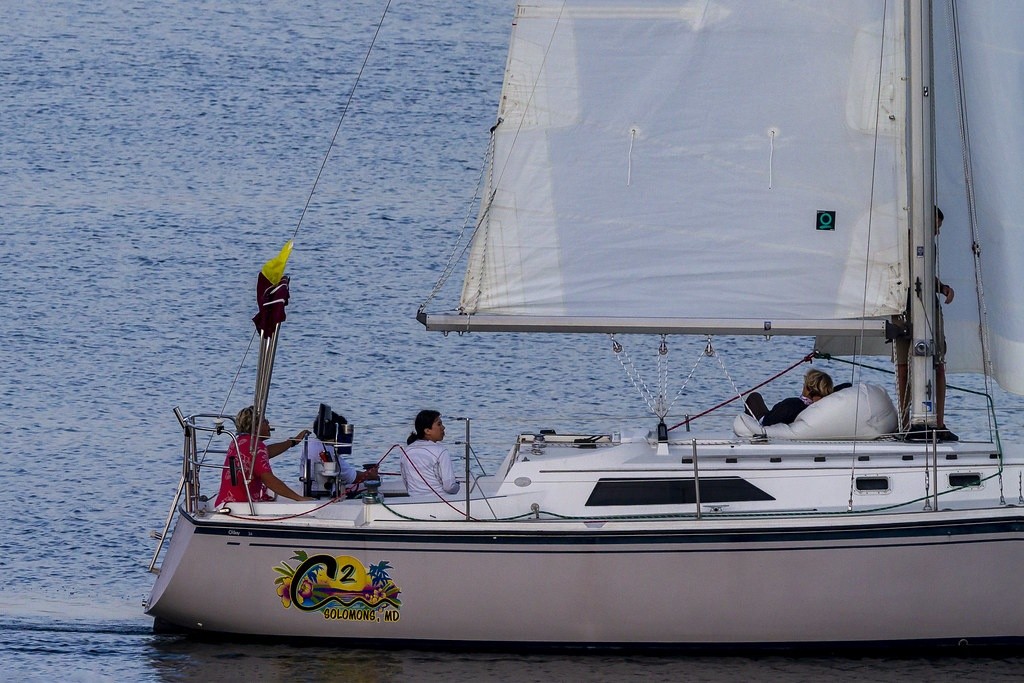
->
[214,405,316,508]
[300,411,380,499]
[744,369,852,427]
[400,410,461,499]
[889,204,959,444]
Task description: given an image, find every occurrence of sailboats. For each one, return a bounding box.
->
[142,0,1022,642]
